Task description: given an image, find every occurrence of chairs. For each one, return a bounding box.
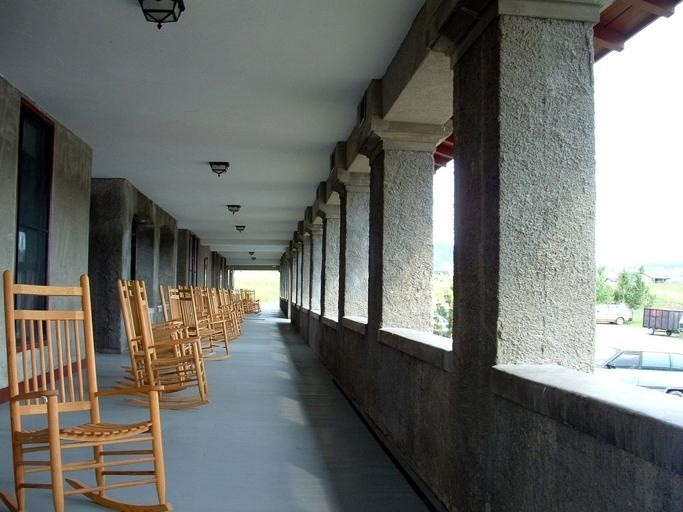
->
[1,270,176,510]
[125,279,209,410]
[177,284,262,362]
[114,278,193,391]
[120,279,208,381]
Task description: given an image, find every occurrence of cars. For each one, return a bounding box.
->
[595,341,683,398]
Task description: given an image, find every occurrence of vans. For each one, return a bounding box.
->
[595,304,632,325]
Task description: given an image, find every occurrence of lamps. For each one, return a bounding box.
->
[227,204,241,214]
[139,1,185,30]
[209,161,229,178]
[235,225,245,232]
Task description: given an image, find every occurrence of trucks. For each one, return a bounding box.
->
[643,307,683,336]
